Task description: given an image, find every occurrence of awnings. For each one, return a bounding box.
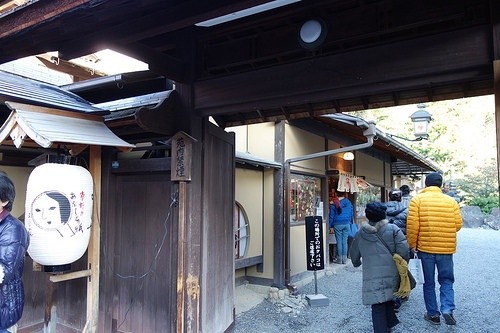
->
[288,112,440,174]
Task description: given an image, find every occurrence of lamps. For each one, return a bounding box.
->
[343,151,354,160]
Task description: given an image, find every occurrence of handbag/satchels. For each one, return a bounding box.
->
[408,253,426,285]
[348,217,359,238]
[398,268,416,291]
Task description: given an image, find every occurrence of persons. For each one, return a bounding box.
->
[406,172,463,325]
[399,184,413,199]
[0,169,30,333]
[384,188,408,235]
[350,202,410,333]
[329,186,353,264]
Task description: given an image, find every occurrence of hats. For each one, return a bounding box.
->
[364,201,388,223]
[425,172,442,188]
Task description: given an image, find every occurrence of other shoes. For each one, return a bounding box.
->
[423,313,441,325]
[333,257,342,263]
[443,313,457,325]
[342,255,347,263]
